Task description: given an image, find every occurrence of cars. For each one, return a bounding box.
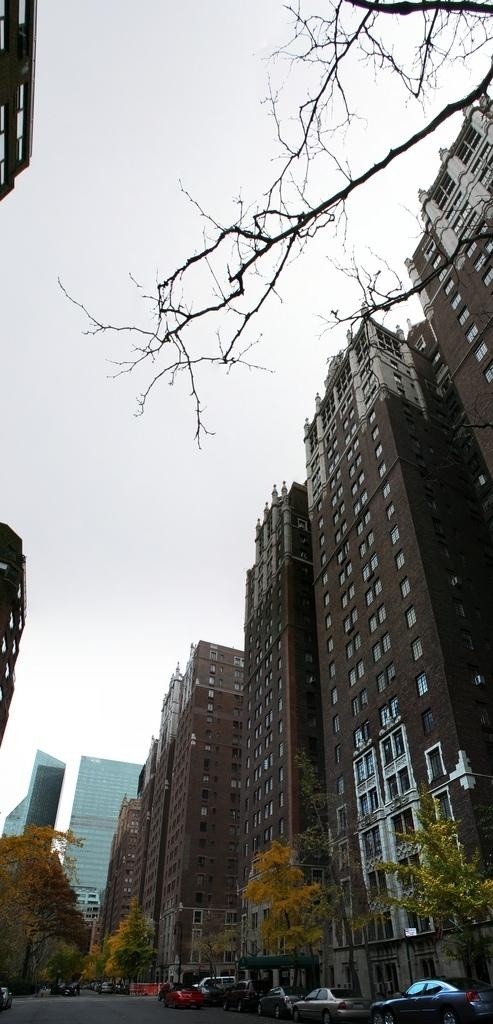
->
[157,983,236,1009]
[221,980,271,1013]
[255,985,310,1020]
[0,987,12,1010]
[290,987,371,1024]
[369,977,492,1024]
[83,981,113,993]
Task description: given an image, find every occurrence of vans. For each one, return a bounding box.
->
[193,975,237,991]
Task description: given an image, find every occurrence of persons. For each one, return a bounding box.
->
[159,981,170,1002]
[76,984,80,996]
